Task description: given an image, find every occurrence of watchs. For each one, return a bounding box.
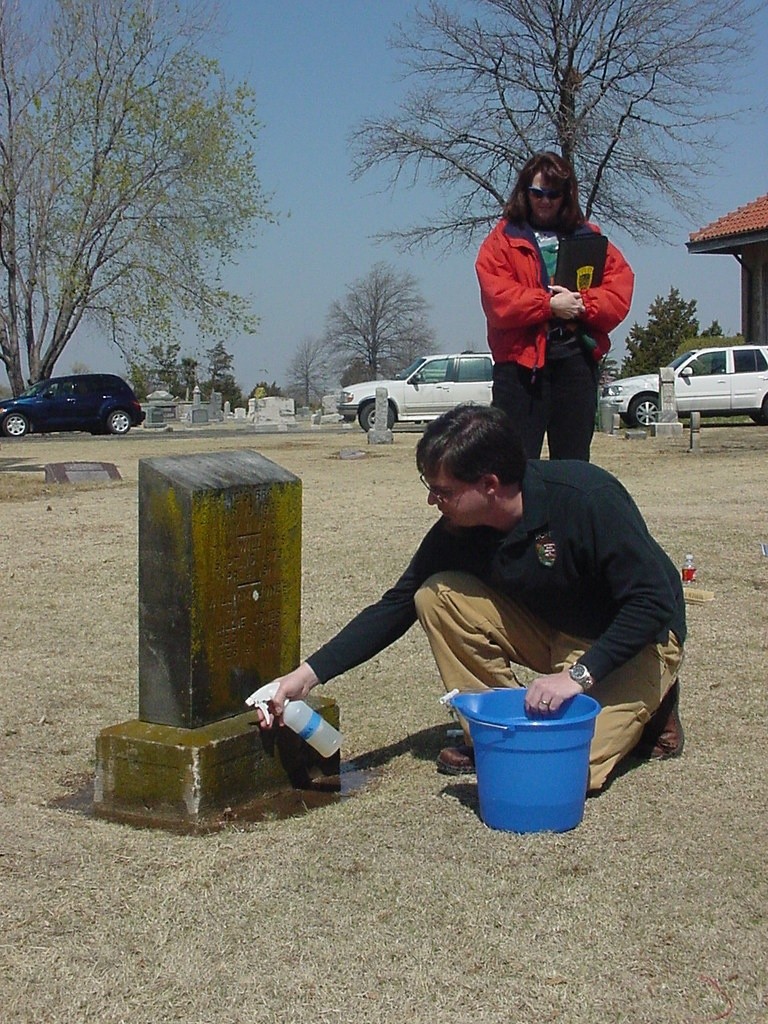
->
[569,661,594,692]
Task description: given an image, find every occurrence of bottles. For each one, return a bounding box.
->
[682,555,697,584]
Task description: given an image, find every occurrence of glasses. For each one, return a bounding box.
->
[420,473,483,503]
[526,186,567,199]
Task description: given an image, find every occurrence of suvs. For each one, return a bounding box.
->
[602,344,768,430]
[336,349,495,433]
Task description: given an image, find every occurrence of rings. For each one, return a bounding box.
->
[539,700,549,706]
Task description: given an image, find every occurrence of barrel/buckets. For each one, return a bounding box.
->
[439,687,602,833]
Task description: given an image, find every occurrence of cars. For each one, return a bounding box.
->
[0,373,147,437]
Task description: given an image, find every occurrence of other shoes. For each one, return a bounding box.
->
[638,676,685,758]
[436,741,477,774]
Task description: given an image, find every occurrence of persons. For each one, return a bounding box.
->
[474,151,635,462]
[257,406,686,791]
